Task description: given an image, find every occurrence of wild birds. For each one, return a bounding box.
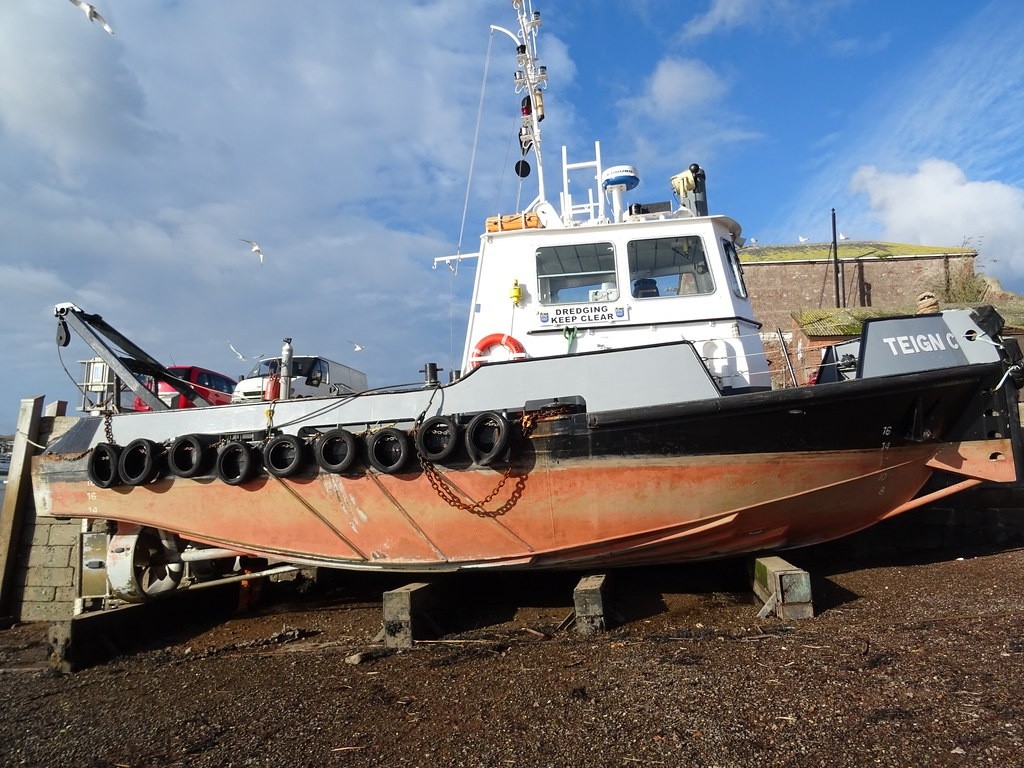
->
[750,236,759,246]
[240,237,264,265]
[346,339,372,351]
[839,232,851,244]
[799,234,810,244]
[227,339,265,361]
[69,0,119,38]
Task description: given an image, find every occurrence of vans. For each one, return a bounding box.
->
[134,365,238,412]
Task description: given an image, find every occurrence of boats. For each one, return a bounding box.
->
[31,0,1024,598]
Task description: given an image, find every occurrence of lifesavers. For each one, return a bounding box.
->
[315,428,358,473]
[263,433,306,478]
[118,437,156,485]
[86,441,118,488]
[469,326,525,369]
[215,441,253,486]
[416,415,459,464]
[368,427,411,474]
[167,433,205,481]
[463,410,511,467]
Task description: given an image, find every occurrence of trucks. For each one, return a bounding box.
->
[230,355,367,403]
